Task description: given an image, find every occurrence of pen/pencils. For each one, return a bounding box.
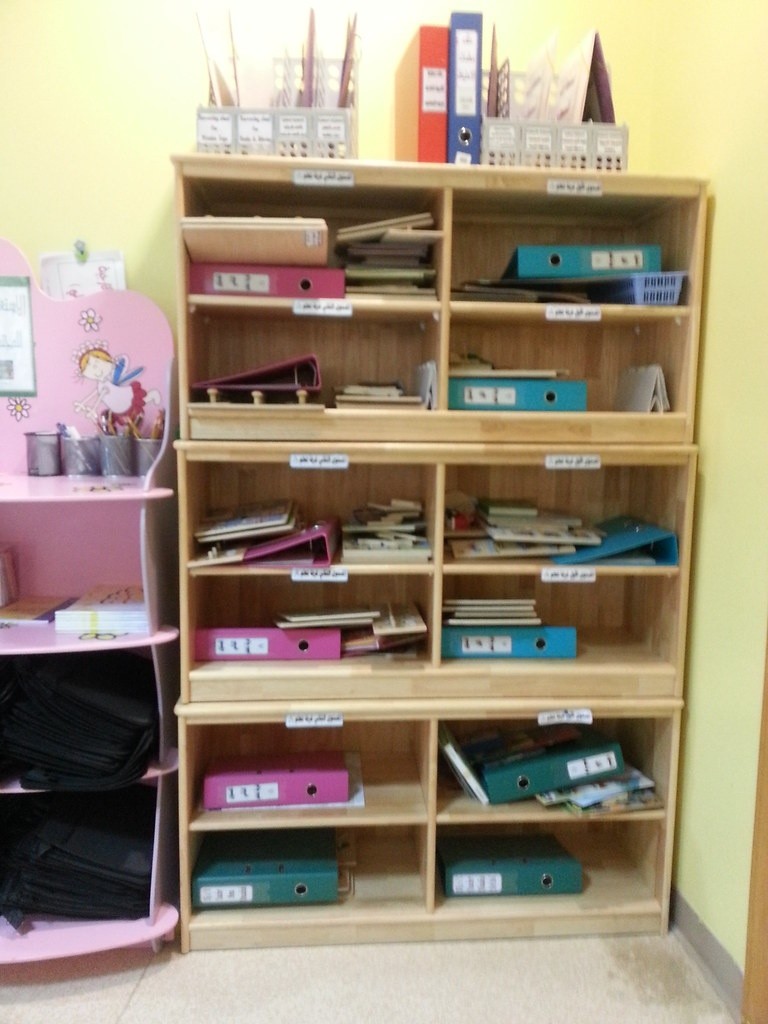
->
[91,407,129,477]
[127,408,166,461]
[54,420,94,476]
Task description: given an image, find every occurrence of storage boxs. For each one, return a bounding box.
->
[197,108,358,160]
[482,118,627,172]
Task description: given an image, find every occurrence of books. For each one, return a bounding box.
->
[0,594,79,624]
[341,502,432,565]
[442,598,541,625]
[55,583,148,633]
[332,383,424,410]
[448,358,557,377]
[475,499,601,545]
[336,211,444,301]
[438,722,489,804]
[194,498,299,542]
[272,601,427,650]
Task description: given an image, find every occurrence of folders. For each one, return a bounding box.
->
[435,829,583,898]
[391,13,482,162]
[185,257,346,300]
[482,728,628,803]
[188,354,323,392]
[445,377,591,409]
[204,514,342,568]
[195,621,343,662]
[201,747,350,808]
[500,244,662,285]
[191,828,340,911]
[552,512,681,566]
[440,623,578,660]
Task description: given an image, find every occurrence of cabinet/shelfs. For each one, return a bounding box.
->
[177,154,713,952]
[0,237,178,965]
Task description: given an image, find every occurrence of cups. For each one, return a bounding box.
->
[132,437,161,477]
[97,435,136,476]
[23,432,62,476]
[62,435,100,476]
